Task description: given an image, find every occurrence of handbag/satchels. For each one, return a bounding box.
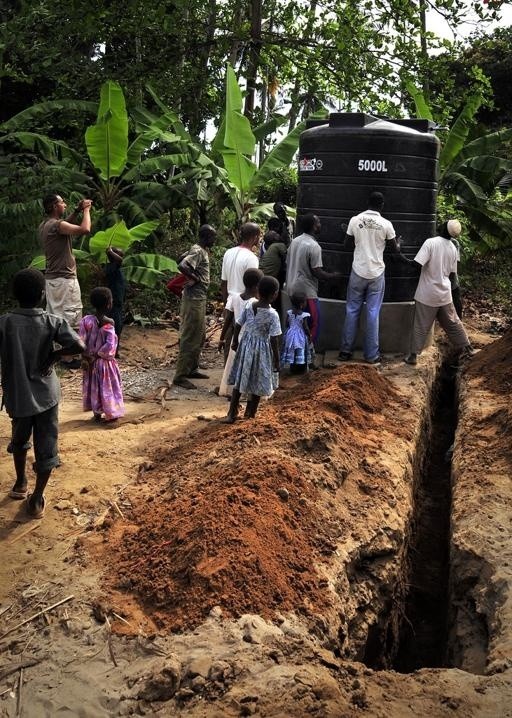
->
[166,272,186,299]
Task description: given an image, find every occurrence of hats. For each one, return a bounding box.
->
[446,218,462,237]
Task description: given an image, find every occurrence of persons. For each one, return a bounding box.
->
[0,266,89,519]
[279,291,313,376]
[337,191,405,365]
[100,245,124,359]
[172,223,218,388]
[38,192,94,367]
[214,201,297,424]
[435,236,463,325]
[77,286,126,428]
[393,216,476,366]
[285,212,342,354]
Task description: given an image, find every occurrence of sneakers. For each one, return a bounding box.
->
[337,350,354,362]
[63,357,81,369]
[367,355,384,363]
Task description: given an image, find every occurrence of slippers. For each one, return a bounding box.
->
[26,490,48,520]
[8,478,30,499]
